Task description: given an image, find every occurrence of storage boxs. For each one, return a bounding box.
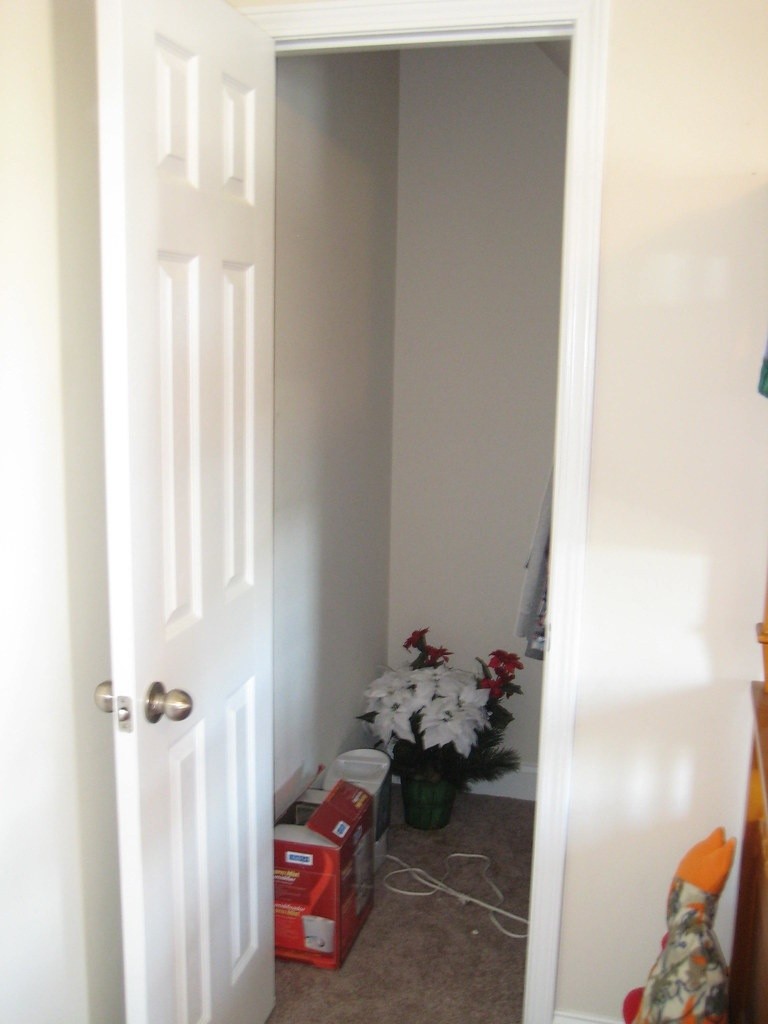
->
[274,760,376,970]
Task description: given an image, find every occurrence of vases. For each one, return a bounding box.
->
[400,747,455,831]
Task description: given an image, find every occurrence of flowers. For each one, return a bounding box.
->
[355,626,524,793]
